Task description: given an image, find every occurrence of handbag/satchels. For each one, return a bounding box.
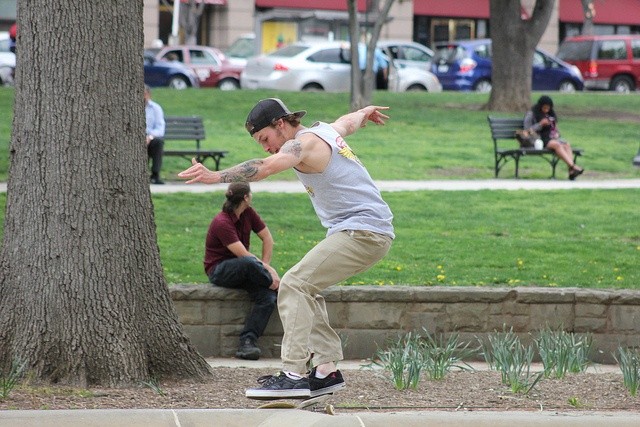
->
[516,131,541,148]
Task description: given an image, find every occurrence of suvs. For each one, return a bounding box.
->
[555,34,640,93]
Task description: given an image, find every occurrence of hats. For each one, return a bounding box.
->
[244,98,306,136]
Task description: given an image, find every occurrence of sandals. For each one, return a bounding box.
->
[569,164,583,180]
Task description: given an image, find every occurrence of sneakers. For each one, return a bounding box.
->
[308,367,346,398]
[246,371,311,399]
[236,336,261,360]
[151,177,164,184]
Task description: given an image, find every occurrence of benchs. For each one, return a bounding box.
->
[487,115,584,178]
[148,117,229,171]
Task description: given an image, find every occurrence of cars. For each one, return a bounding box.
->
[428,38,585,93]
[0,30,16,86]
[376,39,435,71]
[240,37,442,93]
[155,44,244,91]
[144,49,199,91]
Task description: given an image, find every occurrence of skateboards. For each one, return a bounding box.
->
[259,392,334,415]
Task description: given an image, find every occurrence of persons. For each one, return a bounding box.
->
[177,97,395,401]
[143,82,166,184]
[355,33,386,96]
[521,94,585,179]
[204,180,281,359]
